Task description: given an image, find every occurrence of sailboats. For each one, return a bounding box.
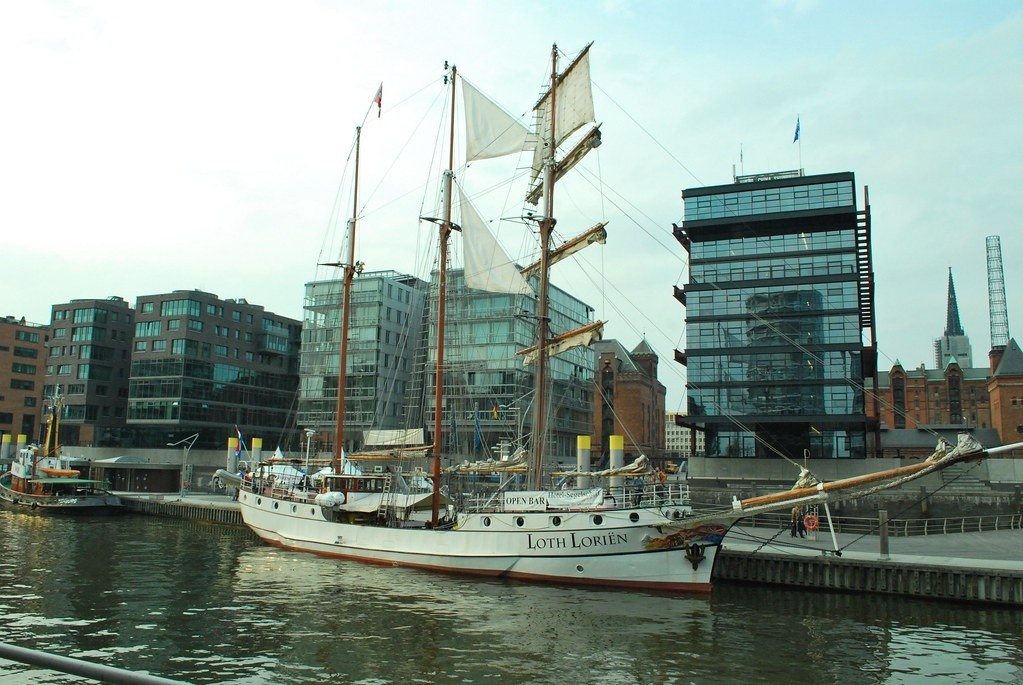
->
[237,37,1023,595]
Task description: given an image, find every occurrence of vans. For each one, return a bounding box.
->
[665,464,680,474]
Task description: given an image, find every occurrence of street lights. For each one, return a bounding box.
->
[302,431,315,492]
[165,432,200,498]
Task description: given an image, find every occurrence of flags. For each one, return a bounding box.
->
[234,428,242,460]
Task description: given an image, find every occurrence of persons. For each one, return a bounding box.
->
[672,508,680,518]
[791,505,807,539]
[654,466,667,505]
[182,479,189,496]
[632,475,645,505]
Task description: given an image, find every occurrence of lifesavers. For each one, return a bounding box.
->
[804,516,818,530]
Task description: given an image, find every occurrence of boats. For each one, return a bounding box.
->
[0,382,129,517]
[215,468,244,489]
[39,467,80,479]
